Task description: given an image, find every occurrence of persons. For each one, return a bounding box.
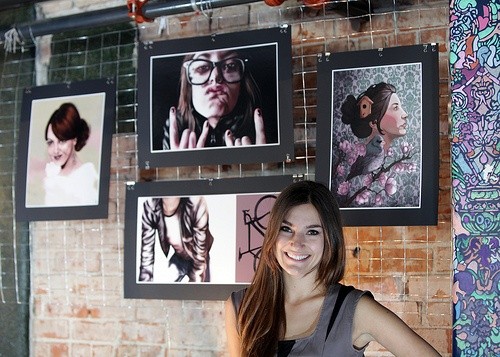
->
[162,48,270,149]
[138,196,214,284]
[44,102,100,207]
[224,181,446,357]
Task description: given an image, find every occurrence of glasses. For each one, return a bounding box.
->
[182,56,249,84]
